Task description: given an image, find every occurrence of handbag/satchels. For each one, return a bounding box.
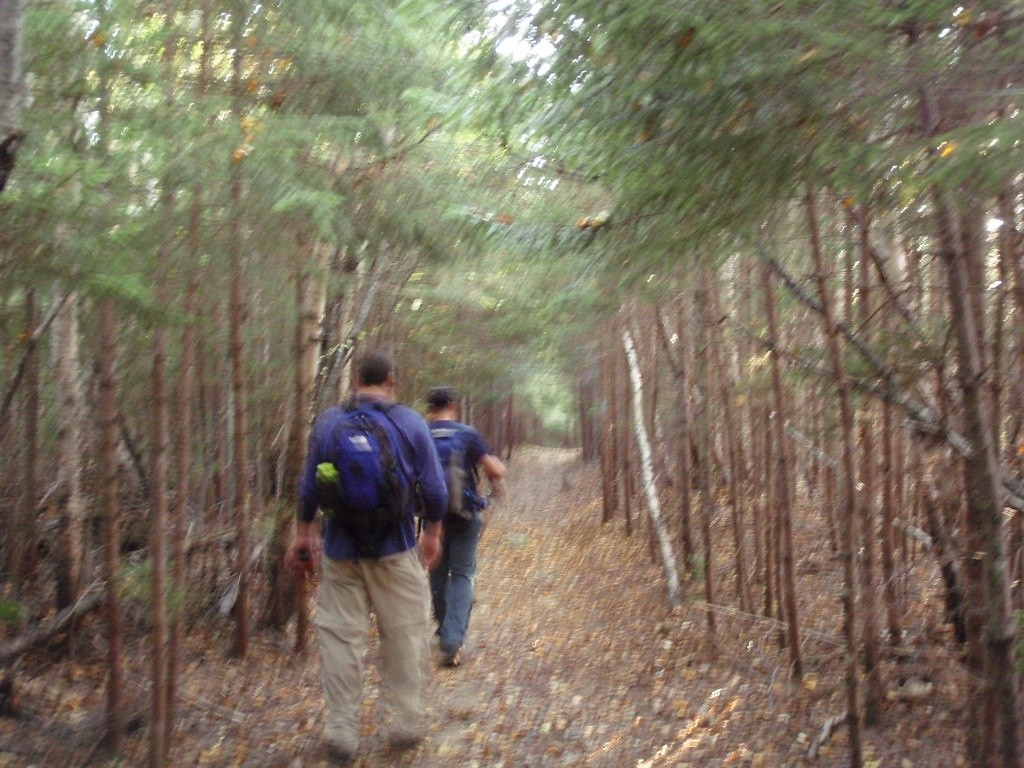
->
[425,423,479,523]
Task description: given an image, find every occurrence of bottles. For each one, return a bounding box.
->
[293,546,315,596]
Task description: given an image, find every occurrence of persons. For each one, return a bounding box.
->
[289,351,449,766]
[422,388,508,666]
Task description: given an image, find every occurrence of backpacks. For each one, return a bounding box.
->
[313,397,418,544]
